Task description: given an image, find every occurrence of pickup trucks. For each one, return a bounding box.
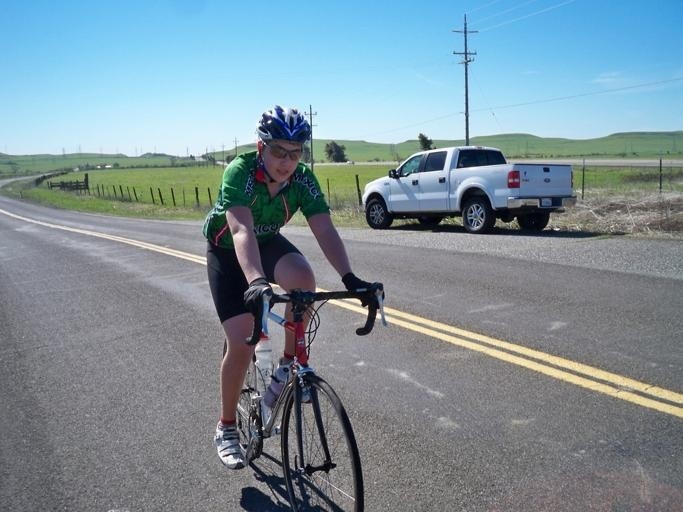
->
[362,146,577,234]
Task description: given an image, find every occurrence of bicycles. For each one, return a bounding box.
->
[221,285,388,511]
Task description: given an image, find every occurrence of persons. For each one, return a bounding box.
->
[199,104,386,470]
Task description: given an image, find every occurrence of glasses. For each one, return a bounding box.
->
[263,142,303,161]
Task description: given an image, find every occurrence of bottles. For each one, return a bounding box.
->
[264,367,290,407]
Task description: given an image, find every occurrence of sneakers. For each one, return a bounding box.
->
[277,359,317,403]
[213,422,245,471]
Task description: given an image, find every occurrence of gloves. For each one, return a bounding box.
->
[244,277,274,318]
[342,270,385,309]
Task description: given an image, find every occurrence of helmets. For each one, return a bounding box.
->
[256,105,312,145]
